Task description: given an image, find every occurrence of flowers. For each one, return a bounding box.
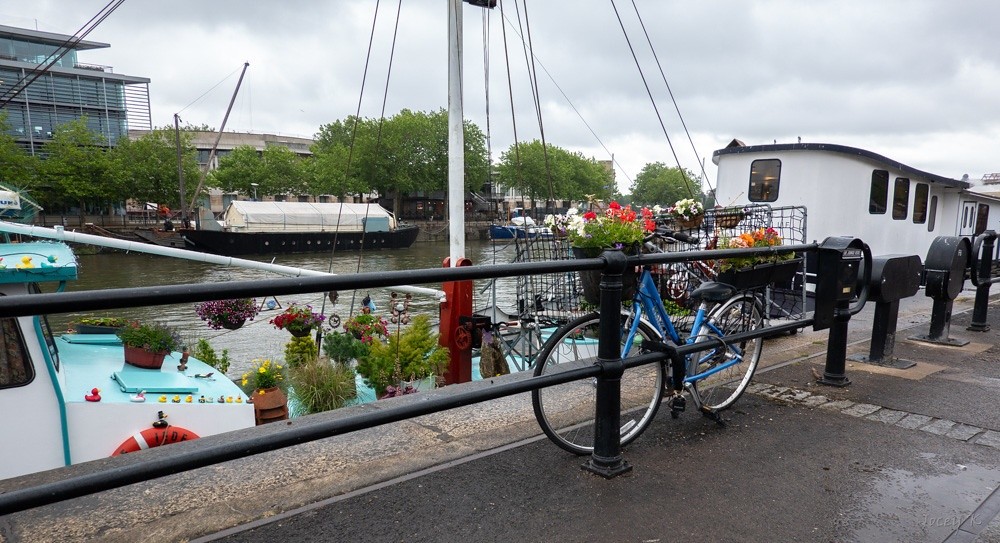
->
[194,297,259,332]
[114,317,182,355]
[546,201,656,247]
[712,205,750,214]
[667,198,704,217]
[343,314,388,344]
[242,359,288,394]
[715,227,795,274]
[268,303,325,331]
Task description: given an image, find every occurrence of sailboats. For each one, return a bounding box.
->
[282,0,738,421]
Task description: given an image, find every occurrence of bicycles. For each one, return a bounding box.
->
[526,229,767,458]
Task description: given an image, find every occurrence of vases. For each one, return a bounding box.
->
[290,329,311,337]
[353,333,362,339]
[552,228,567,239]
[676,214,703,228]
[715,214,744,228]
[573,247,636,306]
[124,344,165,369]
[223,320,245,330]
[250,387,288,426]
[717,255,805,291]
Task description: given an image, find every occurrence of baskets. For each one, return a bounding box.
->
[572,241,632,303]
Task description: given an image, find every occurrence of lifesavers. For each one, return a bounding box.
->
[112,425,200,457]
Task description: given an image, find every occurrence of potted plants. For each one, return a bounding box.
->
[374,321,450,400]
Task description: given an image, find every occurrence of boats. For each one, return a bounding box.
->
[174,198,423,257]
[0,182,258,481]
[488,215,569,242]
